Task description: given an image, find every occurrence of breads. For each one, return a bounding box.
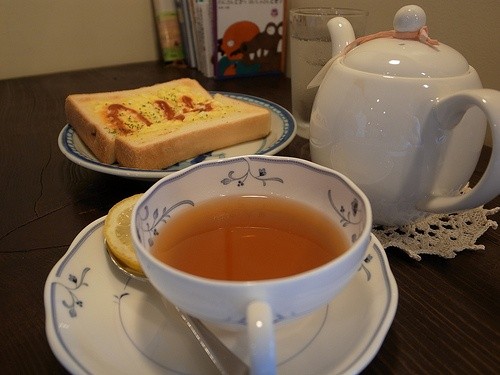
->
[65,78,272,168]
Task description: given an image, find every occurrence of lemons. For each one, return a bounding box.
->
[104,192,142,273]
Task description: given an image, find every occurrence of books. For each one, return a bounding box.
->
[154,0,292,79]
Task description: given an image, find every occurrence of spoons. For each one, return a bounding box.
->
[104,235,251,375]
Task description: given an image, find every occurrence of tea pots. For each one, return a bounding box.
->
[305,3,500,224]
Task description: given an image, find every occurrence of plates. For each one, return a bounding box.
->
[57,91,298,182]
[40,183,398,374]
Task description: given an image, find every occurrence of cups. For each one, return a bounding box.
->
[130,156,373,375]
[287,7,371,141]
